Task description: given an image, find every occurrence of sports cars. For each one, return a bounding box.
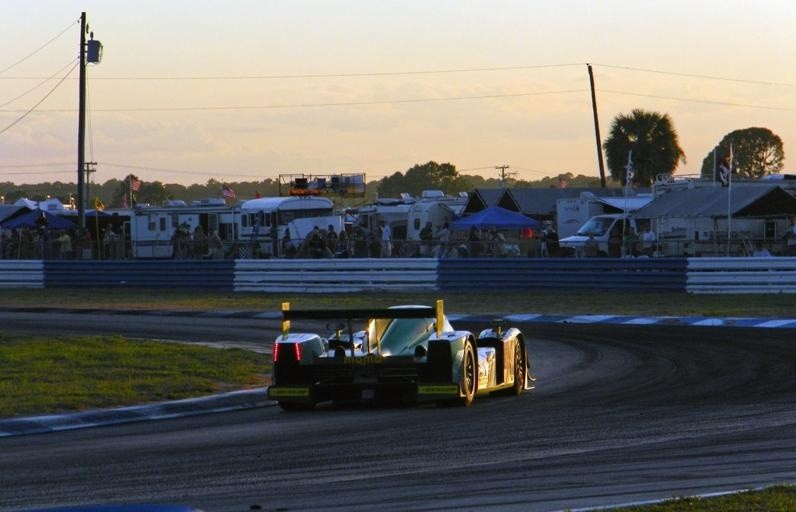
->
[266,298,537,411]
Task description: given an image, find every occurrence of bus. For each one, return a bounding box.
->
[240,196,334,257]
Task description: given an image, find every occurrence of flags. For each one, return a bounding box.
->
[223,184,236,199]
[716,148,731,188]
[129,176,143,191]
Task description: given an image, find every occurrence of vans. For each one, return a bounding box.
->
[558,214,672,256]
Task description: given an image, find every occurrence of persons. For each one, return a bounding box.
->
[752,240,773,258]
[582,230,600,258]
[782,215,796,257]
[0,224,224,260]
[639,220,658,256]
[627,225,641,256]
[292,216,396,259]
[609,226,622,256]
[418,220,559,258]
